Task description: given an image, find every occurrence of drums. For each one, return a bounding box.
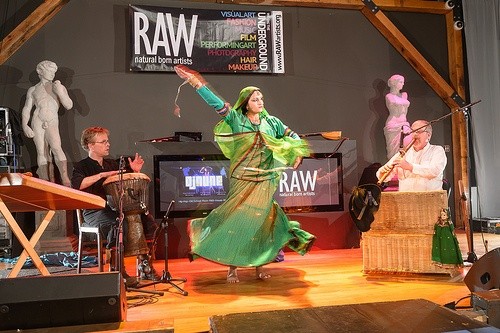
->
[103,173,152,257]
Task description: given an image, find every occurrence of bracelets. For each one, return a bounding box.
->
[188,74,199,87]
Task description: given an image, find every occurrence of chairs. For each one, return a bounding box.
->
[76,209,112,272]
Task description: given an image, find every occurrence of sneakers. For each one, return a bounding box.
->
[120,272,137,285]
[141,272,161,281]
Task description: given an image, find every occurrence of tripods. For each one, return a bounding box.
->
[118,167,164,296]
[136,200,188,296]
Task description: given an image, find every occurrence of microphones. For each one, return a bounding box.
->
[120,156,126,167]
[400,126,404,148]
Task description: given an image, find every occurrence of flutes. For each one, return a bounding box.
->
[377,138,416,186]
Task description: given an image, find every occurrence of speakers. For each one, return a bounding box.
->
[0,270,127,331]
[463,247,500,293]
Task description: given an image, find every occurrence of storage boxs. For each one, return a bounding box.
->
[472,218,500,233]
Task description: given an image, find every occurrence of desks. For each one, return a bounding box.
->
[0,172,106,277]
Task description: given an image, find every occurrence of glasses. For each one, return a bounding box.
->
[88,140,109,145]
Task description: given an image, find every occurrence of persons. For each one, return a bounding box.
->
[71,126,161,287]
[384,75,412,160]
[171,65,317,283]
[376,120,447,192]
[22,61,73,189]
